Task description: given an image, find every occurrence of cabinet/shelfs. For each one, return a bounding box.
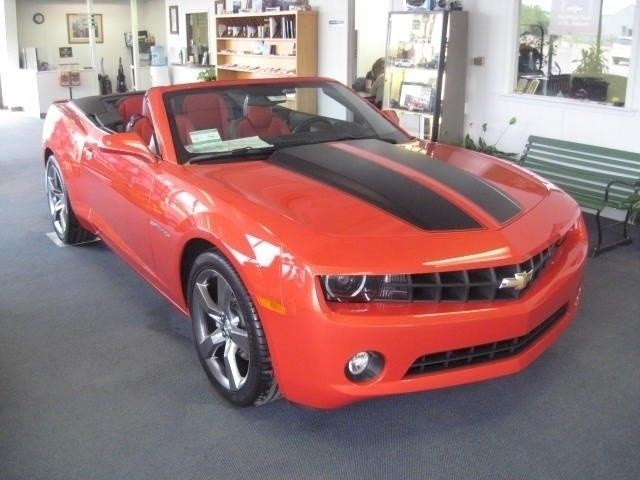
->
[383,9,467,146]
[214,10,317,114]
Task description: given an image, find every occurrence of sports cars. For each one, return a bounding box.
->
[42,76,590,409]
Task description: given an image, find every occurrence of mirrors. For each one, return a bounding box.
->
[184,11,209,64]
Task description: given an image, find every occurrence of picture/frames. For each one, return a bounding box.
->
[168,5,179,34]
[67,13,104,44]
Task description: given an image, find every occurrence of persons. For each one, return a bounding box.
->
[365,57,385,110]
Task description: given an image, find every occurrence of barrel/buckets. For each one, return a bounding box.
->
[150,46,166,64]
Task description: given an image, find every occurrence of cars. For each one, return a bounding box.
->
[611,37,632,65]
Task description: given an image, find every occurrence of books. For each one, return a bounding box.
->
[217,16,296,76]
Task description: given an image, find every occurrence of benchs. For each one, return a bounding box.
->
[515,134,639,260]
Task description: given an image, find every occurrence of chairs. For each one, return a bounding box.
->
[116,92,292,147]
